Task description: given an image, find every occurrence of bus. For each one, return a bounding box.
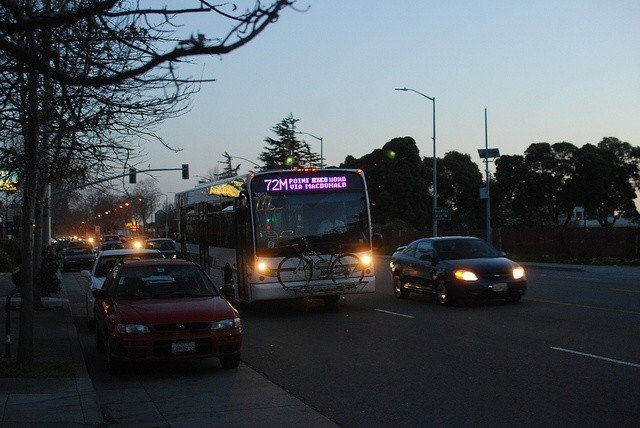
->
[175,169,376,302]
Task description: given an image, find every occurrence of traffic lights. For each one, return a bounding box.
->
[182,165,189,180]
[129,168,136,182]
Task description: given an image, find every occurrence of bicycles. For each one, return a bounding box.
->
[277,236,365,290]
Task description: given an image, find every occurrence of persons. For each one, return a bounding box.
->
[315,204,348,234]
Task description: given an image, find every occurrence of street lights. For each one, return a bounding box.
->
[81,198,144,230]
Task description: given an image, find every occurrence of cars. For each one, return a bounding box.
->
[91,259,243,375]
[86,249,168,320]
[390,236,527,305]
[64,234,176,270]
